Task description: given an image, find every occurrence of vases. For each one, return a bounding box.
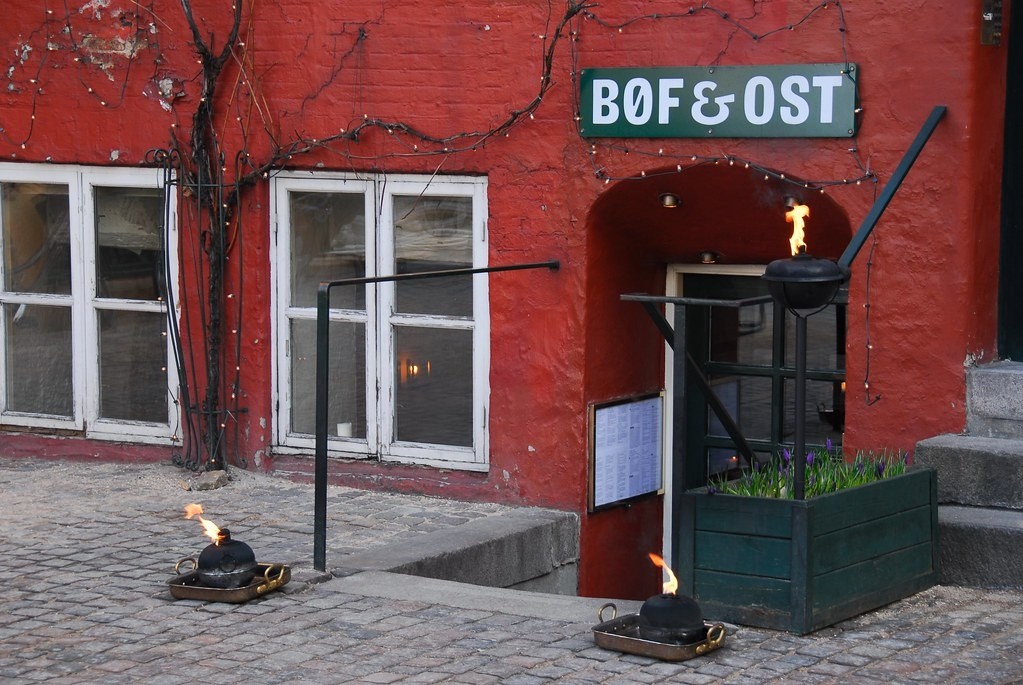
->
[680,465,942,638]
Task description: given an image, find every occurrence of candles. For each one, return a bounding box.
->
[337,422,353,438]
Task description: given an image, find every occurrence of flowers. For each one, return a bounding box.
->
[707,439,911,498]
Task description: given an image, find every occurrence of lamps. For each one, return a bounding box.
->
[702,253,714,264]
[661,196,677,208]
[786,197,801,210]
[407,359,420,383]
